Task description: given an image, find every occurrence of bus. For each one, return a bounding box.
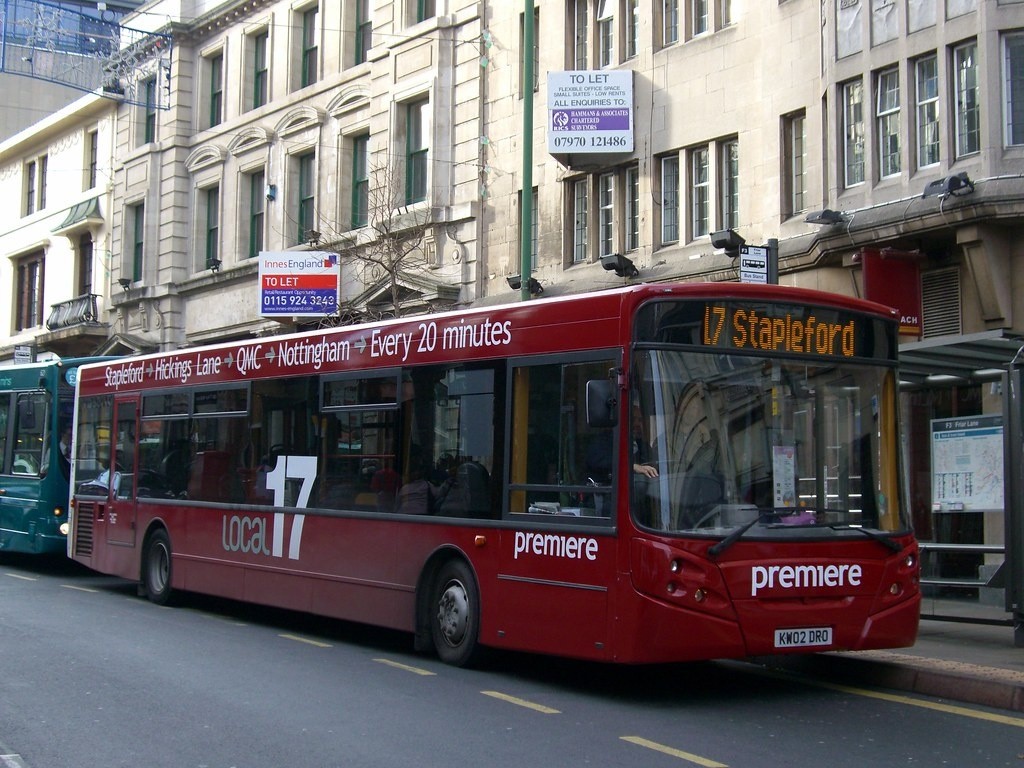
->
[0,355,143,555]
[65,280,920,666]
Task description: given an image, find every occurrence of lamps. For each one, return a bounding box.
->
[598,253,640,276]
[206,258,222,273]
[305,230,322,247]
[803,209,845,226]
[921,172,976,203]
[118,279,133,291]
[506,275,544,293]
[711,230,746,258]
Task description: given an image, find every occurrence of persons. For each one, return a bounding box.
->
[42,426,72,476]
[589,405,659,478]
[323,453,495,514]
[653,393,714,474]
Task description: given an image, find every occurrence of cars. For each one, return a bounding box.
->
[78,470,176,501]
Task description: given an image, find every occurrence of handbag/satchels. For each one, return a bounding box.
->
[425,480,438,514]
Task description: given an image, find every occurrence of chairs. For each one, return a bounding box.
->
[117,449,497,521]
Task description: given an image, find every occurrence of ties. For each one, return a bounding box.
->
[65,447,71,459]
